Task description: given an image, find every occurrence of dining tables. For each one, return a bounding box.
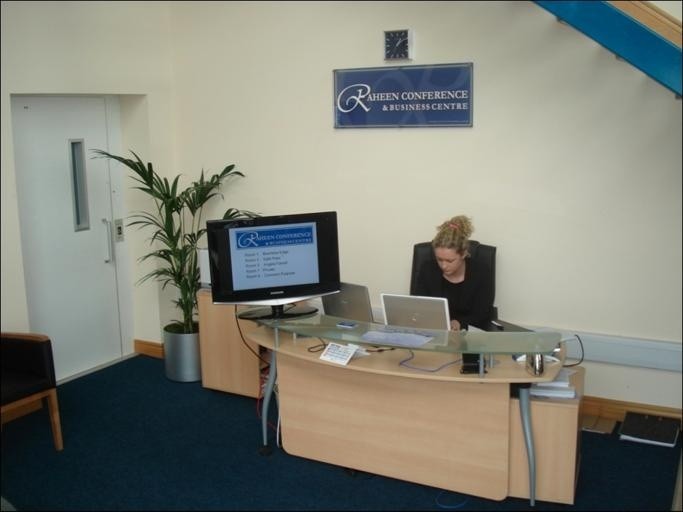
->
[89,148,260,383]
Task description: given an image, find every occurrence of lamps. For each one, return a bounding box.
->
[0,331,64,452]
[409,241,502,332]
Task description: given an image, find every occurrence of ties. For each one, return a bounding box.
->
[206,211,340,320]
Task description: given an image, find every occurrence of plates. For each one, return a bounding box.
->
[509,366,584,505]
[198,289,339,399]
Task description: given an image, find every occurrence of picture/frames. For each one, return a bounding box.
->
[242,312,566,502]
[567,358,683,435]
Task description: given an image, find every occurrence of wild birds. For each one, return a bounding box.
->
[321,281,374,323]
[380,293,452,330]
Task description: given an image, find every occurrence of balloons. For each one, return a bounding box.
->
[383,29,413,60]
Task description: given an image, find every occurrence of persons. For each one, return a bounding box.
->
[413,215,494,334]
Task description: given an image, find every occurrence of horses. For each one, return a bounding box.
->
[336,320,358,328]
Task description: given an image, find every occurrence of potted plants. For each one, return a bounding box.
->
[333,62,474,128]
